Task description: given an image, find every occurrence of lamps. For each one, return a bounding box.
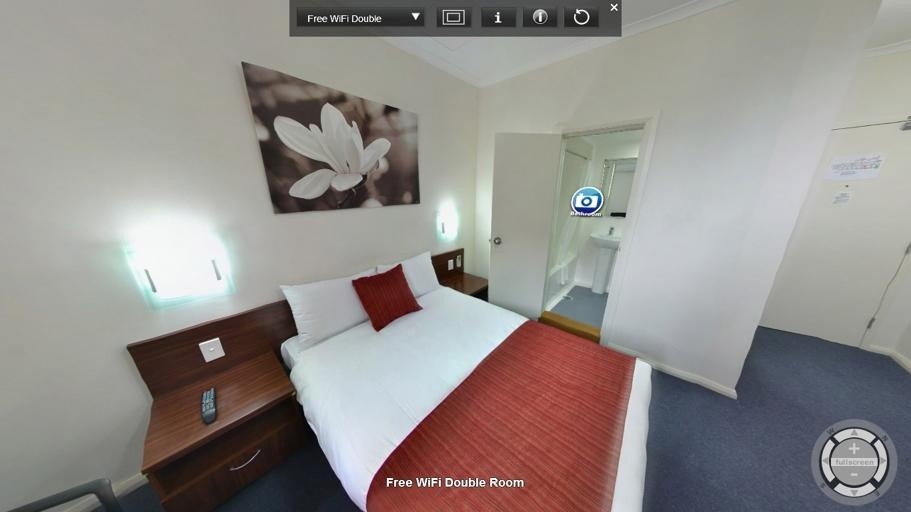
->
[433,219,459,244]
[125,234,237,312]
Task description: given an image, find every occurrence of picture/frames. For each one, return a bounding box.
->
[238,59,426,215]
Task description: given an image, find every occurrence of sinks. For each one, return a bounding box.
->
[590,233,620,250]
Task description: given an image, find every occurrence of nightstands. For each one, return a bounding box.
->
[446,270,489,301]
[140,354,319,511]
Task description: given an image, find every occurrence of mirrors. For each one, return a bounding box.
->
[599,159,638,221]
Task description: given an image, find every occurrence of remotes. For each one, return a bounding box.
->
[201,387,216,425]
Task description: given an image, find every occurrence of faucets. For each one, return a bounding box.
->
[609,227,614,234]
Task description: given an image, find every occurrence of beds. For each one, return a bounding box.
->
[279,251,655,512]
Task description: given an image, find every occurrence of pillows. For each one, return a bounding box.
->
[352,264,424,333]
[377,250,440,299]
[282,269,379,349]
